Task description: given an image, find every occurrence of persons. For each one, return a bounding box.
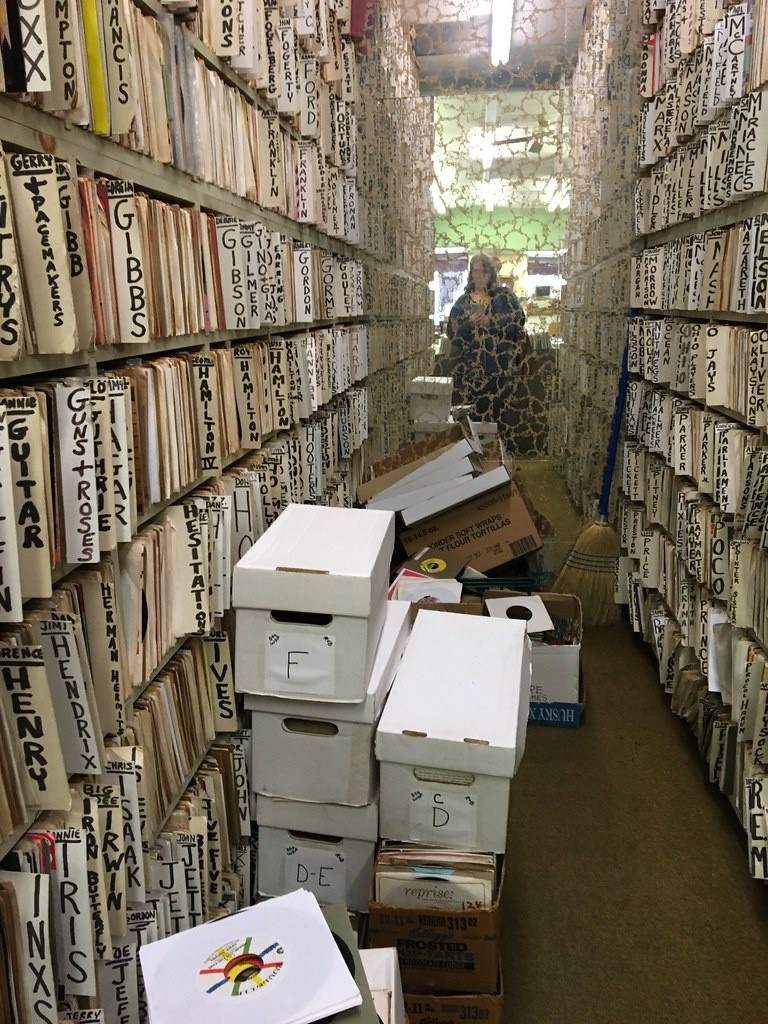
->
[447,253,549,458]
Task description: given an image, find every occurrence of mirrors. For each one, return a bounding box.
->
[355,0,642,595]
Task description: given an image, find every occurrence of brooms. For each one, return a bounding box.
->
[549,308,637,625]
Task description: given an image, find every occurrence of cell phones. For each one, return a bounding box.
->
[472,304,484,316]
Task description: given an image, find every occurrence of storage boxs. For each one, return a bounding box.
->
[135,376,586,1024]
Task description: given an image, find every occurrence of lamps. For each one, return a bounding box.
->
[490,0,514,66]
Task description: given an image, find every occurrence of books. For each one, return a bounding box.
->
[554,0,768,898]
[0,0,436,1024]
